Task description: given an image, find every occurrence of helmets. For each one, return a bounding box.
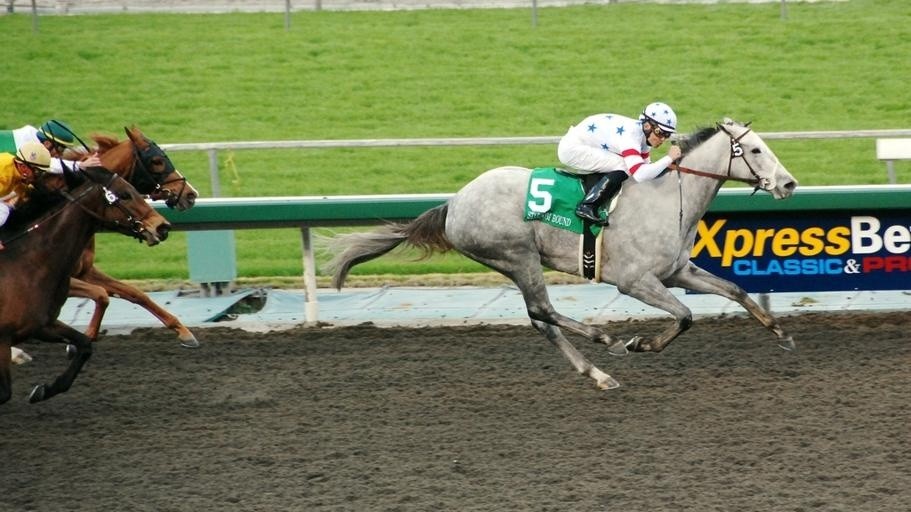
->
[13,142,53,171]
[641,101,676,133]
[36,119,74,146]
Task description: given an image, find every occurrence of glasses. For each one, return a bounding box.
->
[51,139,66,153]
[651,126,671,138]
[27,164,43,179]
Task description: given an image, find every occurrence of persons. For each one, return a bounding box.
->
[0,142,51,250]
[0,118,102,174]
[557,102,682,226]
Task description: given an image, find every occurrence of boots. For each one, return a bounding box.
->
[575,176,622,227]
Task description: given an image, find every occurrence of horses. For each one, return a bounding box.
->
[0,155,172,405]
[309,114,800,392]
[11,123,202,366]
